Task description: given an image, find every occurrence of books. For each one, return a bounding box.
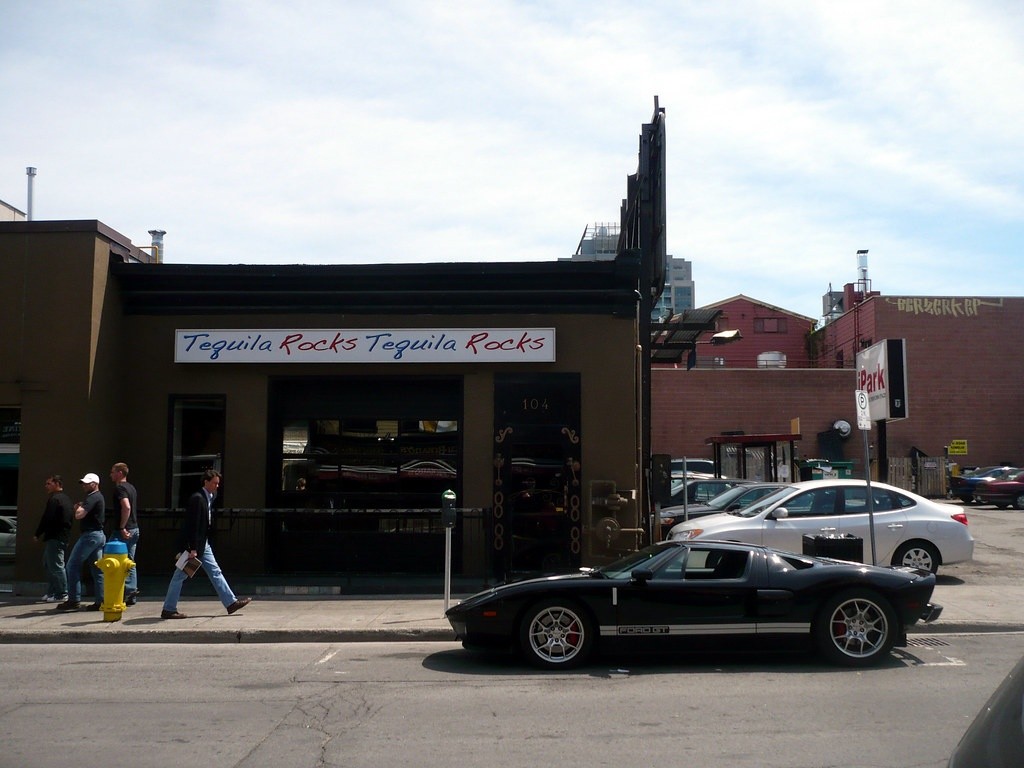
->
[174,551,203,578]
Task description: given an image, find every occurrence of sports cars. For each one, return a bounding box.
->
[445,536,943,670]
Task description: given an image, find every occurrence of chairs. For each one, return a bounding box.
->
[812,492,829,514]
[864,494,876,512]
[876,496,889,512]
[754,489,773,501]
[714,484,732,497]
[712,552,736,580]
[825,491,837,513]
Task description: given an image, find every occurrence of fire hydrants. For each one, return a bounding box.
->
[92,540,137,624]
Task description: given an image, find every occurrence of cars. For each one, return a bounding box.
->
[950,465,1020,505]
[0,515,16,562]
[974,466,1024,509]
[666,478,976,577]
[666,457,757,505]
[645,482,880,548]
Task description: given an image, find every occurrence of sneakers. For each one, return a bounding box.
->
[55,600,80,611]
[41,594,53,600]
[86,602,101,611]
[47,594,68,602]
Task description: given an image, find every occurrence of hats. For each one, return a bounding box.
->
[78,473,99,484]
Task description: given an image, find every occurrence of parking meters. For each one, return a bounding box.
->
[440,489,457,620]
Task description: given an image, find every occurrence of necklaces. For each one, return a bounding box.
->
[88,490,100,496]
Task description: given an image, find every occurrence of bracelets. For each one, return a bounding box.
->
[120,527,126,530]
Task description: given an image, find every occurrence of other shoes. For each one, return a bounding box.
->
[126,600,136,607]
[227,597,251,614]
[161,610,187,619]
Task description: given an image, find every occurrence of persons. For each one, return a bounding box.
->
[57,473,107,612]
[33,476,77,603]
[161,470,253,618]
[103,463,137,605]
